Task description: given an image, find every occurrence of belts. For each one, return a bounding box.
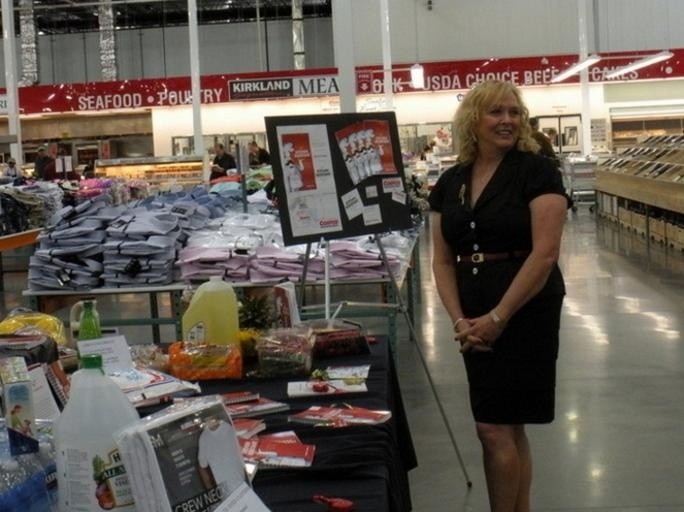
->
[459,249,533,264]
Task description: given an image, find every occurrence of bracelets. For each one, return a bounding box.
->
[452,319,467,328]
[490,311,511,329]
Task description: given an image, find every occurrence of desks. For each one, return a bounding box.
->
[21,232,422,342]
[133,334,417,511]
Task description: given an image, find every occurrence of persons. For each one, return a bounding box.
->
[5,158,22,177]
[430,82,570,512]
[249,141,270,167]
[528,117,561,164]
[34,146,52,177]
[84,158,94,174]
[210,143,236,181]
[196,410,246,501]
[430,141,440,156]
[420,145,431,160]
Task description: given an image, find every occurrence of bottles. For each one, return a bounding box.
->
[181,275,239,349]
[76,301,102,370]
[54,354,143,512]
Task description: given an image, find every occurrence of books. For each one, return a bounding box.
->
[287,401,390,428]
[27,361,71,420]
[174,390,314,468]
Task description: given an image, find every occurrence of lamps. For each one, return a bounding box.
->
[410,64,425,89]
[606,51,674,81]
[549,54,601,84]
